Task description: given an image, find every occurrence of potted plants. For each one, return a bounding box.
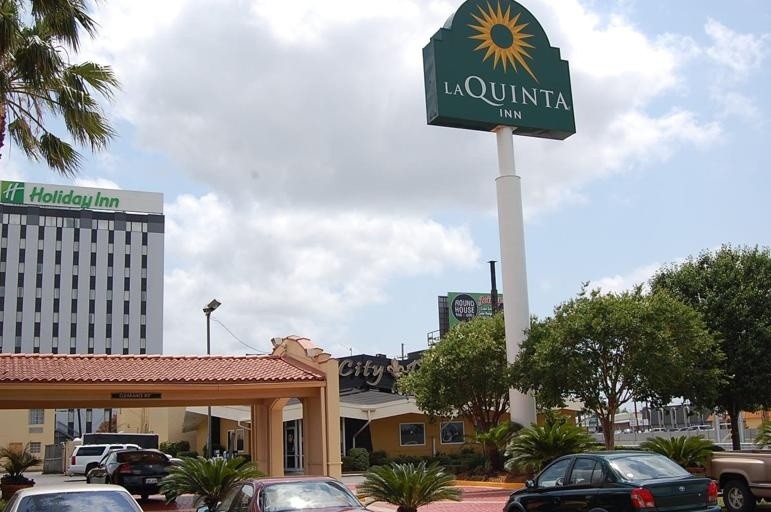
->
[0,440,42,501]
[639,433,727,473]
[355,460,463,512]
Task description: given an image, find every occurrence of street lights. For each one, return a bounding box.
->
[202,299,221,459]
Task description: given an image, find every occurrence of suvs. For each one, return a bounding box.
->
[69,443,141,476]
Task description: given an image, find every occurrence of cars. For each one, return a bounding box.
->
[504,451,720,512]
[594,423,733,436]
[2,482,148,512]
[197,476,376,512]
[87,448,178,506]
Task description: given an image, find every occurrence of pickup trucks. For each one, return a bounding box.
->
[705,449,771,511]
